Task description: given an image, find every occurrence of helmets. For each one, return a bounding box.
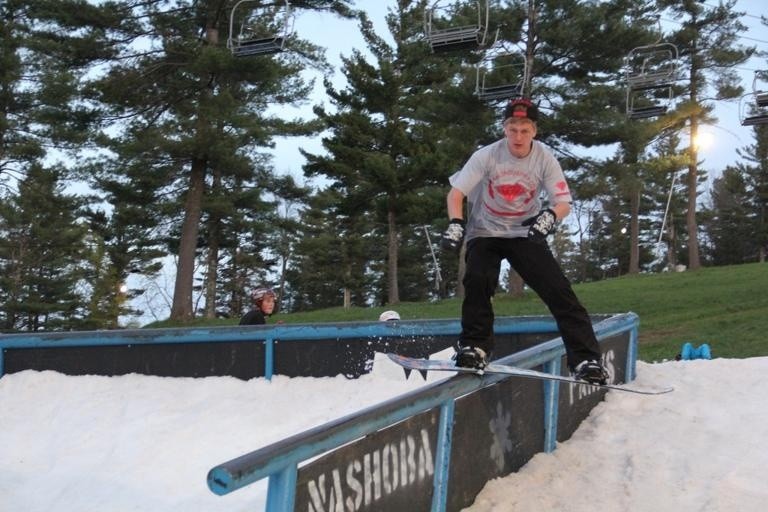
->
[251,287,278,309]
[379,309,403,322]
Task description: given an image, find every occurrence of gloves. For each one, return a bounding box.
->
[441,217,467,252]
[521,208,557,244]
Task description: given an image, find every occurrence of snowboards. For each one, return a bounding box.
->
[387,352,674,395]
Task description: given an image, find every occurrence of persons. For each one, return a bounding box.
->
[442,99,609,388]
[238,288,284,326]
[379,310,401,321]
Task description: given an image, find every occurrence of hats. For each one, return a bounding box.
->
[501,99,542,127]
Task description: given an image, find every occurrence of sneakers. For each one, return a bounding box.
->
[570,358,610,385]
[456,346,492,368]
